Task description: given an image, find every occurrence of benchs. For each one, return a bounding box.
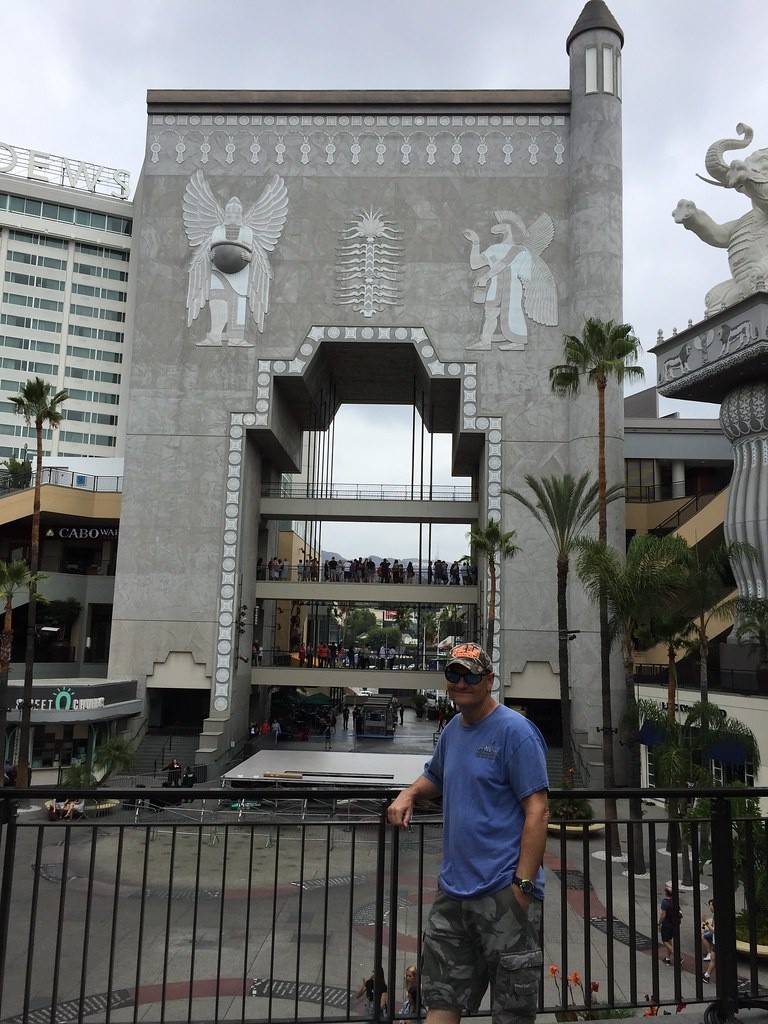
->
[701,919,768,957]
[45,799,118,812]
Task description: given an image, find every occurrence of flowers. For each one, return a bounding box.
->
[547,964,600,1016]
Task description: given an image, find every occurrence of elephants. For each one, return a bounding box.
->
[671,122,768,317]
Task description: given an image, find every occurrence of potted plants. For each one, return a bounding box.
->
[410,693,427,718]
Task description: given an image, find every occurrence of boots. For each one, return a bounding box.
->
[702,953,711,962]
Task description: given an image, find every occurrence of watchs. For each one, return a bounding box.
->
[512,873,535,895]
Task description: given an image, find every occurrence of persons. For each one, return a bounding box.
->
[324,727,331,748]
[386,641,550,1024]
[252,642,263,665]
[659,888,715,982]
[343,705,359,730]
[53,799,84,821]
[256,550,476,585]
[259,721,280,742]
[399,703,404,725]
[300,640,396,669]
[161,759,195,803]
[357,966,426,1023]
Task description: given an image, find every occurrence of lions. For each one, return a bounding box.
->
[658,342,692,384]
[717,320,759,358]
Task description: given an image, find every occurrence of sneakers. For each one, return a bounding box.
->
[680,958,684,965]
[702,972,710,984]
[661,956,672,966]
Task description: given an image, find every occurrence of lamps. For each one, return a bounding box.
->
[241,605,247,610]
[240,612,246,617]
[240,622,245,626]
[240,629,245,634]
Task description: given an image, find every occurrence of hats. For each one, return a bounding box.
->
[443,642,493,675]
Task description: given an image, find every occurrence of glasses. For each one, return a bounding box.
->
[444,671,489,684]
